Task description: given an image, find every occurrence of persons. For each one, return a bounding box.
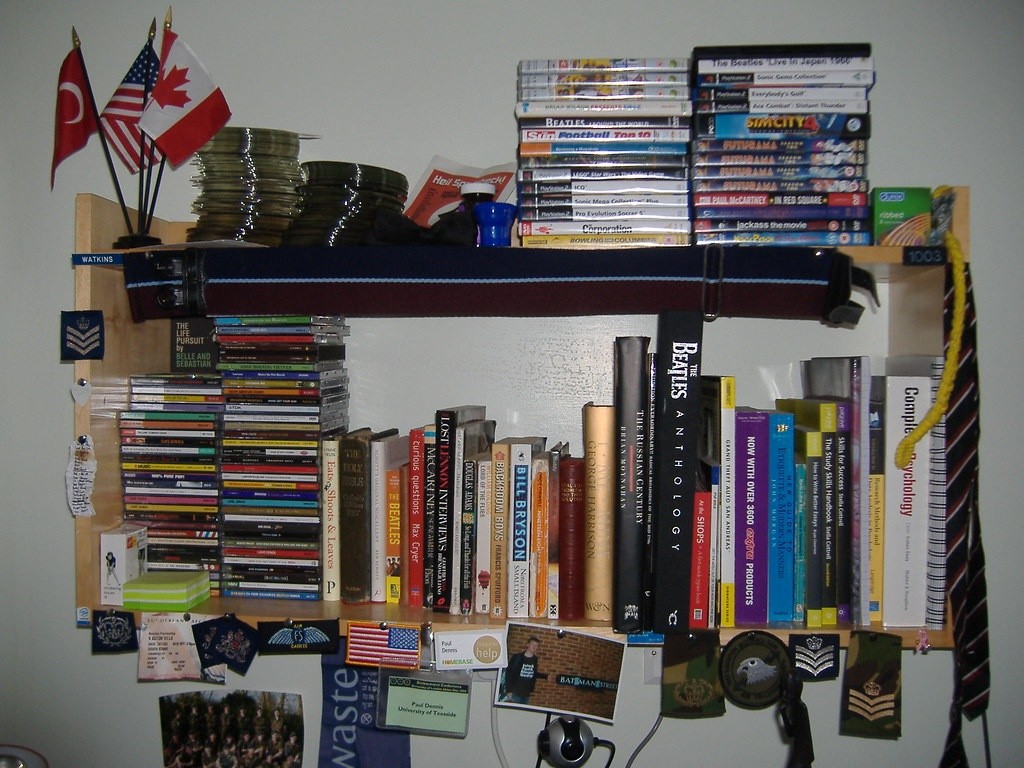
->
[106,552,118,585]
[137,532,146,576]
[506,637,552,705]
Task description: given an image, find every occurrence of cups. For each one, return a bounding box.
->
[475,203,517,247]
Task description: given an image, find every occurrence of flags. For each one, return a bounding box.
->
[99,43,165,175]
[50,47,98,192]
[138,30,233,166]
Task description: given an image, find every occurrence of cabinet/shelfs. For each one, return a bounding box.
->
[73,184,972,649]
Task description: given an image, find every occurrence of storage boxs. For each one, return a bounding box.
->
[868,186,933,247]
[99,521,148,602]
[124,572,211,612]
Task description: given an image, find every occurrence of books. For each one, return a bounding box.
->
[118,314,952,630]
[516,42,878,250]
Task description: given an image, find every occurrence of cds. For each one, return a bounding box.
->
[186,126,410,246]
